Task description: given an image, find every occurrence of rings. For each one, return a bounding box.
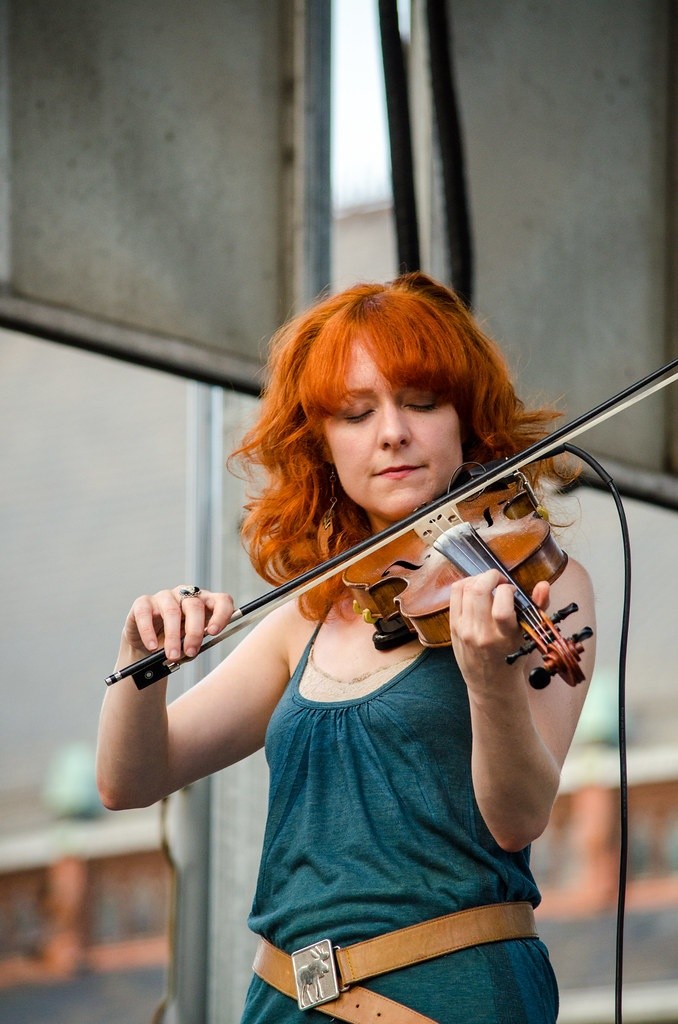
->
[176,585,202,609]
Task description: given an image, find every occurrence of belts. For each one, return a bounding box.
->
[251,902,539,1024]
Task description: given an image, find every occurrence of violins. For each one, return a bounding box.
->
[340,469,596,692]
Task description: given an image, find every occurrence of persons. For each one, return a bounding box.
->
[97,271,598,1024]
